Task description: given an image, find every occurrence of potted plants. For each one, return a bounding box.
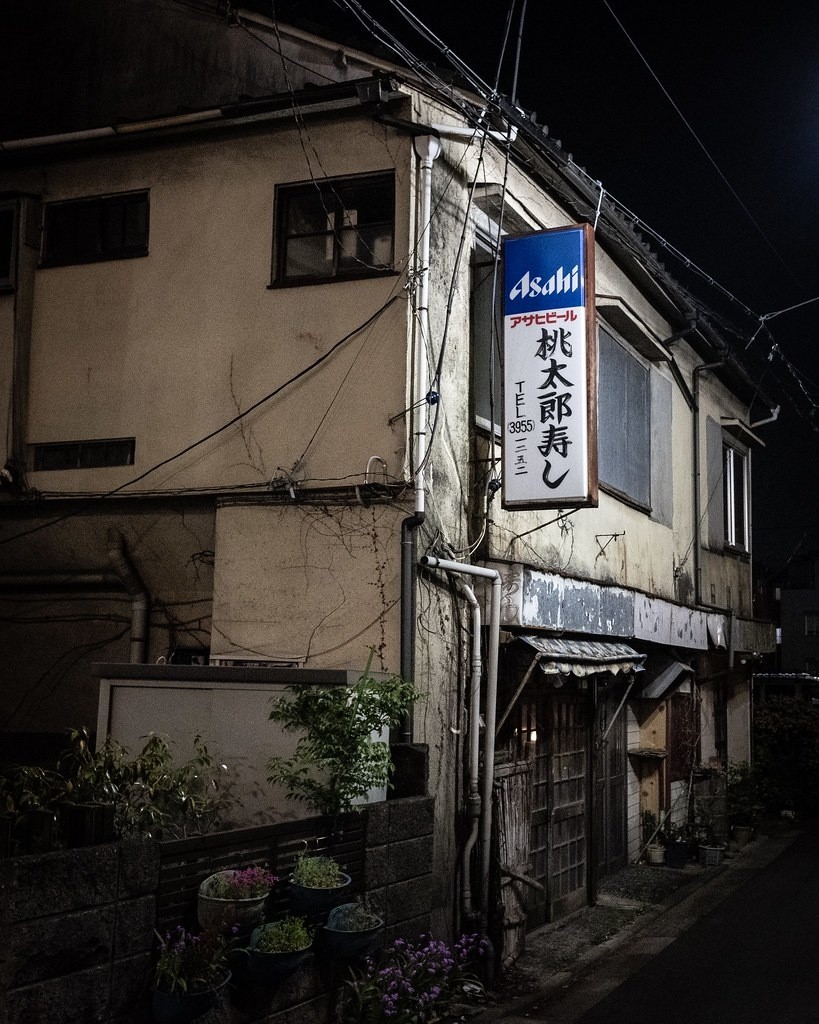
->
[678,776,730,866]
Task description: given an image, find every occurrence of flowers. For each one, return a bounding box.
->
[290,854,345,886]
[215,866,277,898]
[346,930,489,1024]
[250,917,311,948]
[154,923,238,992]
[328,901,379,928]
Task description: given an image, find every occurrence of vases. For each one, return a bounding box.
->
[200,868,269,923]
[247,938,313,976]
[322,917,382,947]
[291,871,352,907]
[154,971,233,1018]
[646,844,665,863]
